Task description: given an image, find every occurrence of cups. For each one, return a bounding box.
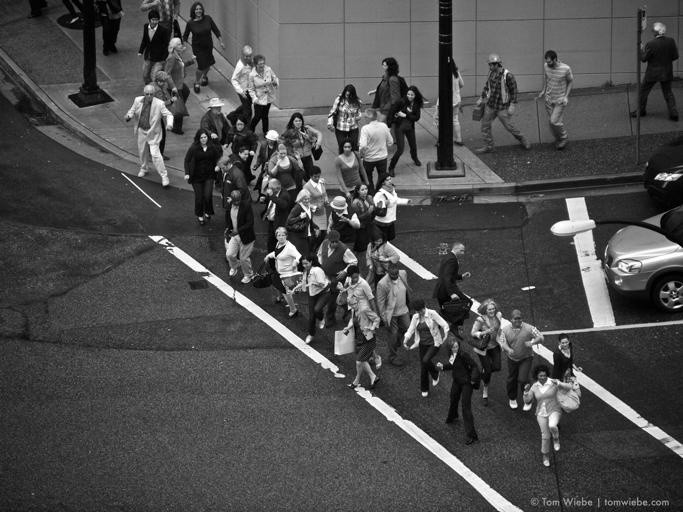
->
[146,92,154,95]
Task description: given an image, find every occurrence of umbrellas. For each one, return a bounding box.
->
[465,433,478,446]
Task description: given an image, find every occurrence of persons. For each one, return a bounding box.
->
[96,0,123,57]
[124,2,579,469]
[629,23,680,122]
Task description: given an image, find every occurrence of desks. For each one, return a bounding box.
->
[546,205,683,249]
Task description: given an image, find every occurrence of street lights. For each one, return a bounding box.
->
[439,299,470,326]
[557,370,582,410]
[311,139,323,160]
[284,203,306,233]
[371,190,388,217]
[253,261,274,288]
[328,96,343,129]
[169,90,190,117]
[334,329,356,356]
[468,316,490,351]
[336,277,349,306]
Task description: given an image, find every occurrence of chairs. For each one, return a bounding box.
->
[542,453,551,467]
[346,355,403,389]
[287,309,326,344]
[173,129,183,134]
[553,438,561,451]
[422,391,429,398]
[412,158,421,167]
[509,399,518,409]
[558,139,569,148]
[193,76,208,93]
[137,171,145,178]
[475,145,496,154]
[482,397,488,407]
[197,215,205,225]
[161,176,169,187]
[522,402,532,412]
[229,260,250,283]
[630,109,646,118]
[389,166,394,177]
[432,371,440,387]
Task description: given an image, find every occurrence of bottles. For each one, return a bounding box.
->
[265,129,279,141]
[328,230,341,241]
[330,196,348,210]
[487,54,502,64]
[207,98,224,108]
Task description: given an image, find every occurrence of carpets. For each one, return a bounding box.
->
[262,201,274,222]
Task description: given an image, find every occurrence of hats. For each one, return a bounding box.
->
[601,204,683,313]
[641,135,683,200]
